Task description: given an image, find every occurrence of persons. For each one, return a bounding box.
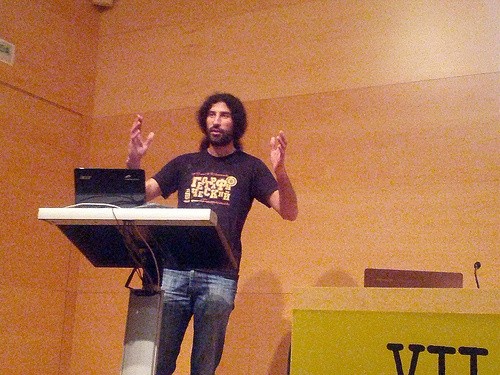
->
[125,92,298,374]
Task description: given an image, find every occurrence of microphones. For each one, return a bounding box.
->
[474,262,481,287]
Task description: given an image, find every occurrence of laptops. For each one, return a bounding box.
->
[74,168,146,208]
[364,268,464,288]
